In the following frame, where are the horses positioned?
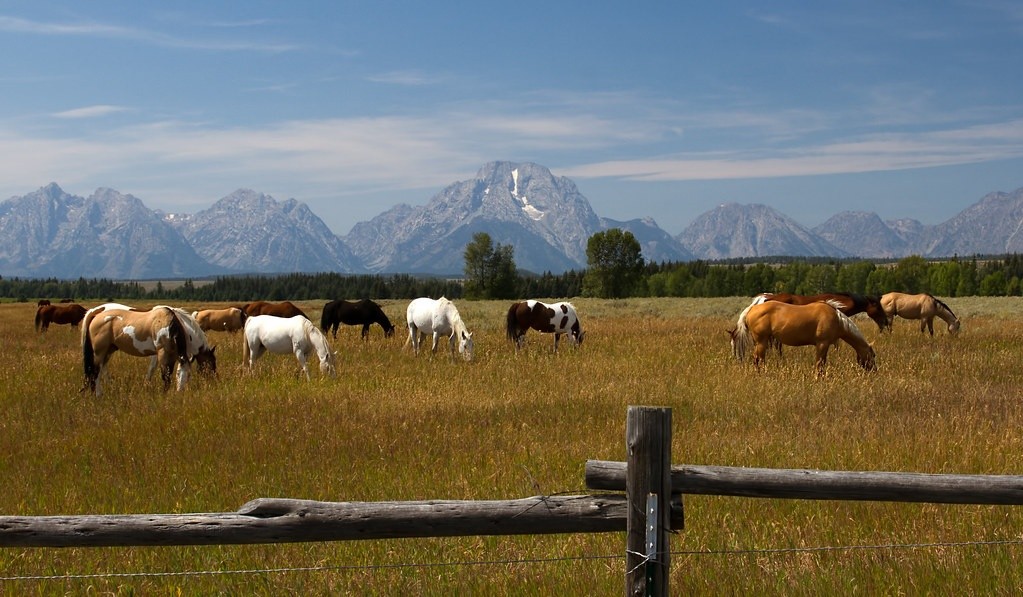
[34,299,395,396]
[507,299,586,352]
[403,297,473,361]
[728,291,961,374]
